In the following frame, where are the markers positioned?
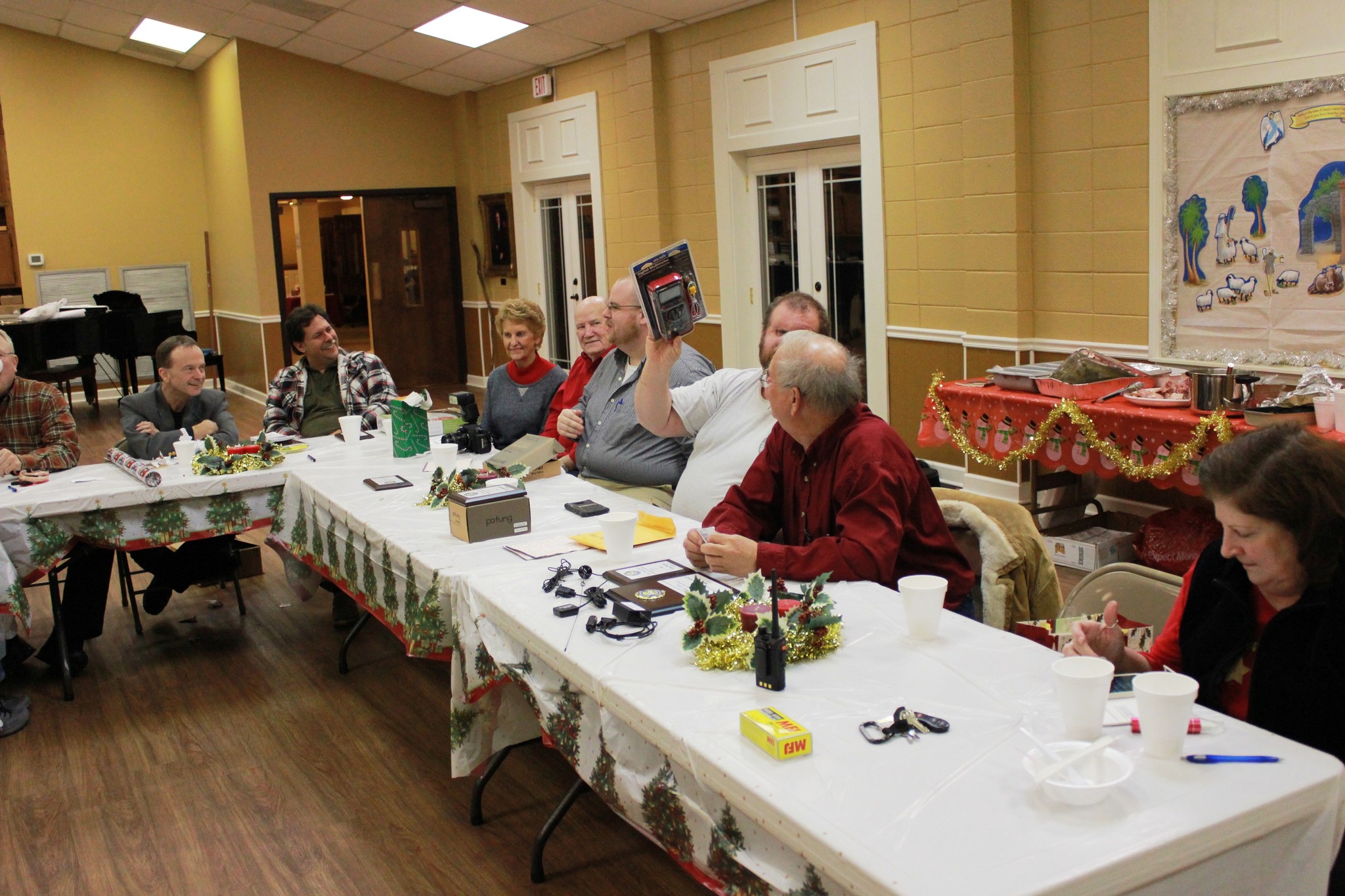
[1131,716,1215,734]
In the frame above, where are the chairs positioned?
[110,436,249,635]
[1057,560,1187,665]
[931,484,1063,645]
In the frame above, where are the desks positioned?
[918,372,1345,504]
[0,426,1345,894]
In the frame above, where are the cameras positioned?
[440,391,491,454]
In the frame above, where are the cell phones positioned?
[1107,671,1137,699]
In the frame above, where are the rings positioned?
[1107,620,1119,629]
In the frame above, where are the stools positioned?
[35,364,99,420]
[199,347,226,396]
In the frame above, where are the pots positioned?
[1185,369,1260,417]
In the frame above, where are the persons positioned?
[263,302,397,623]
[683,335,980,621]
[118,336,240,615]
[635,291,828,522]
[0,329,114,672]
[480,278,719,514]
[1062,420,1345,896]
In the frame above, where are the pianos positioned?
[0,290,197,413]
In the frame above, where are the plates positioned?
[1123,388,1191,407]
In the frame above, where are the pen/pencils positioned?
[1181,755,1284,764]
[307,455,316,462]
[8,486,18,492]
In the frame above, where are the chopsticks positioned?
[1033,737,1121,783]
[1015,724,1095,785]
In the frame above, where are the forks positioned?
[992,365,1004,371]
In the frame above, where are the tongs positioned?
[1091,382,1145,403]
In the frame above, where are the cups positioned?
[599,512,637,563]
[1132,671,1199,760]
[1313,389,1345,434]
[897,575,948,641]
[430,443,458,478]
[1051,656,1115,743]
[338,415,362,442]
[173,440,197,471]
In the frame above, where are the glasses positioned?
[607,303,641,313]
[759,370,807,398]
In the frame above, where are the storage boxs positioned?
[1040,524,1140,573]
[482,434,566,484]
[449,496,531,544]
[389,388,431,457]
[740,708,811,760]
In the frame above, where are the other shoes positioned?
[35,625,90,675]
[3,707,30,737]
[331,590,359,627]
[3,635,37,669]
[0,694,31,712]
[143,564,175,615]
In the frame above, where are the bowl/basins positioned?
[1024,741,1133,806]
